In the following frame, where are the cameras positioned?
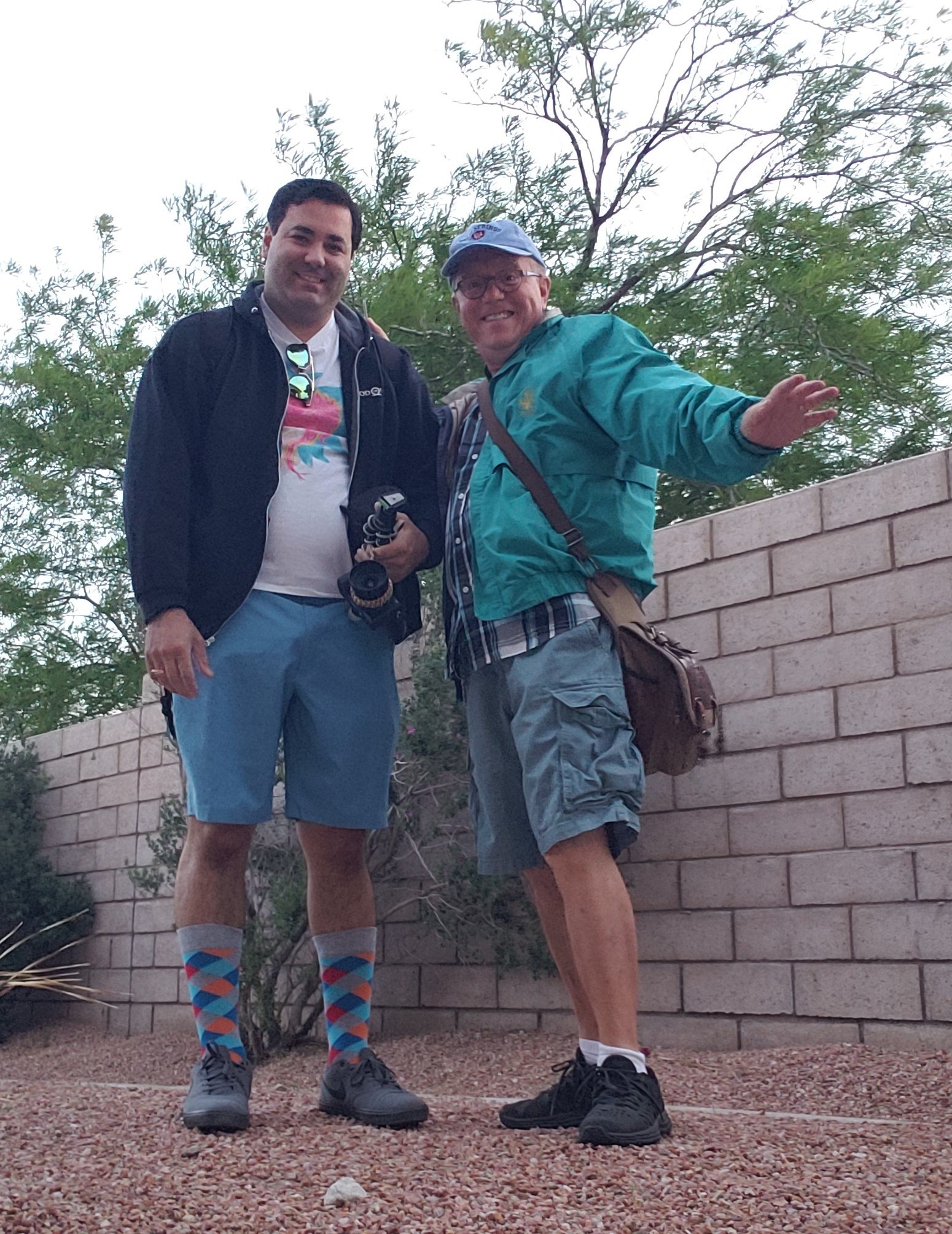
[337,560,410,628]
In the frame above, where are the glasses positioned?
[286,343,315,406]
[454,268,543,300]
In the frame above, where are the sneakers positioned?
[319,1048,429,1125]
[500,1047,599,1129]
[182,1040,253,1129]
[579,1055,672,1144]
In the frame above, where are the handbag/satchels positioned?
[614,622,724,775]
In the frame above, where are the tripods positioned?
[361,491,409,548]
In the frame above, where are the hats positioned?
[441,219,547,277]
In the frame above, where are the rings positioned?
[151,668,164,675]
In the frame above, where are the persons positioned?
[123,177,445,1132]
[367,220,841,1140]
